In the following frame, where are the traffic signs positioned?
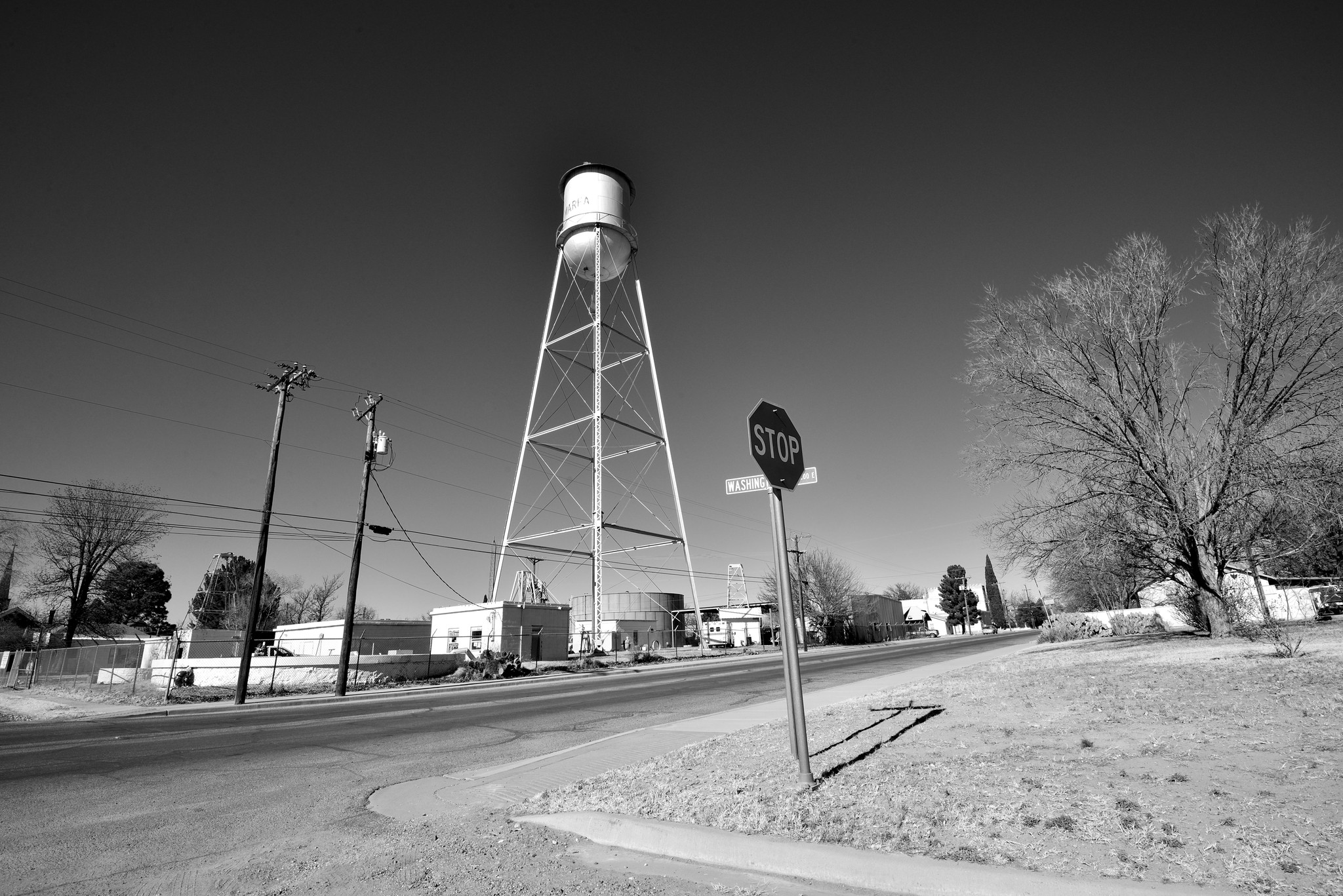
[726,466,819,494]
[1043,597,1055,605]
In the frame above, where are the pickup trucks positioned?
[906,626,939,639]
[252,645,314,657]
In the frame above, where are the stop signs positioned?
[746,397,805,492]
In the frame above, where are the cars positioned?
[982,625,998,635]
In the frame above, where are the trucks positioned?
[701,621,737,648]
